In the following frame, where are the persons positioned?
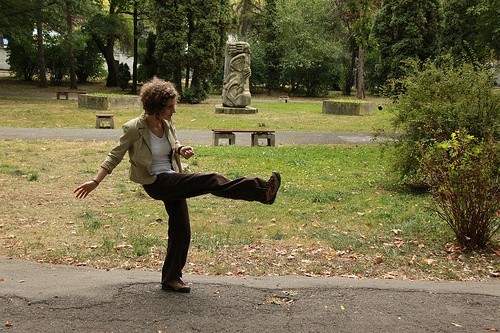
[68,75,282,293]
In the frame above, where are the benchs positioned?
[213,129,276,146]
[57,91,85,100]
[96,113,114,129]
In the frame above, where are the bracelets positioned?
[92,179,99,186]
[178,145,183,155]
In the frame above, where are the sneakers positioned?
[260,171,281,205]
[161,277,191,293]
